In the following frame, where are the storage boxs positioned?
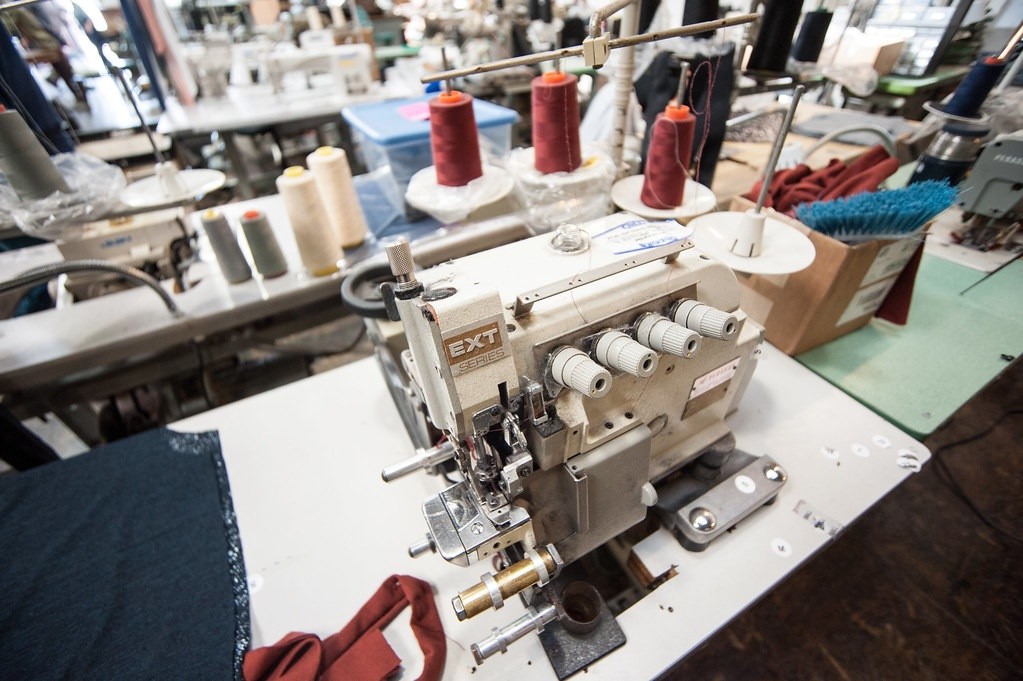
[341,92,519,224]
[56,203,192,263]
[838,29,908,77]
[731,191,932,359]
